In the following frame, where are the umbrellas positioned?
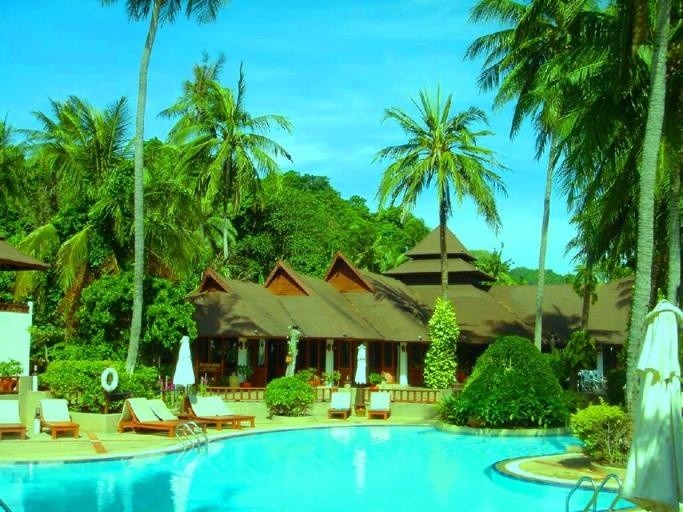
[620,300,682,511]
[354,344,367,385]
[172,335,195,395]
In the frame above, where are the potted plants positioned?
[0,359,24,394]
[235,364,255,391]
[367,372,387,390]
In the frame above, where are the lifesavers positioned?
[102,368,118,392]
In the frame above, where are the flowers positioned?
[286,325,309,356]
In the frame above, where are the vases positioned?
[284,356,292,363]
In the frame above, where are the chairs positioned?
[0,400,26,440]
[327,392,352,419]
[40,399,80,439]
[117,395,256,438]
[368,392,391,419]
